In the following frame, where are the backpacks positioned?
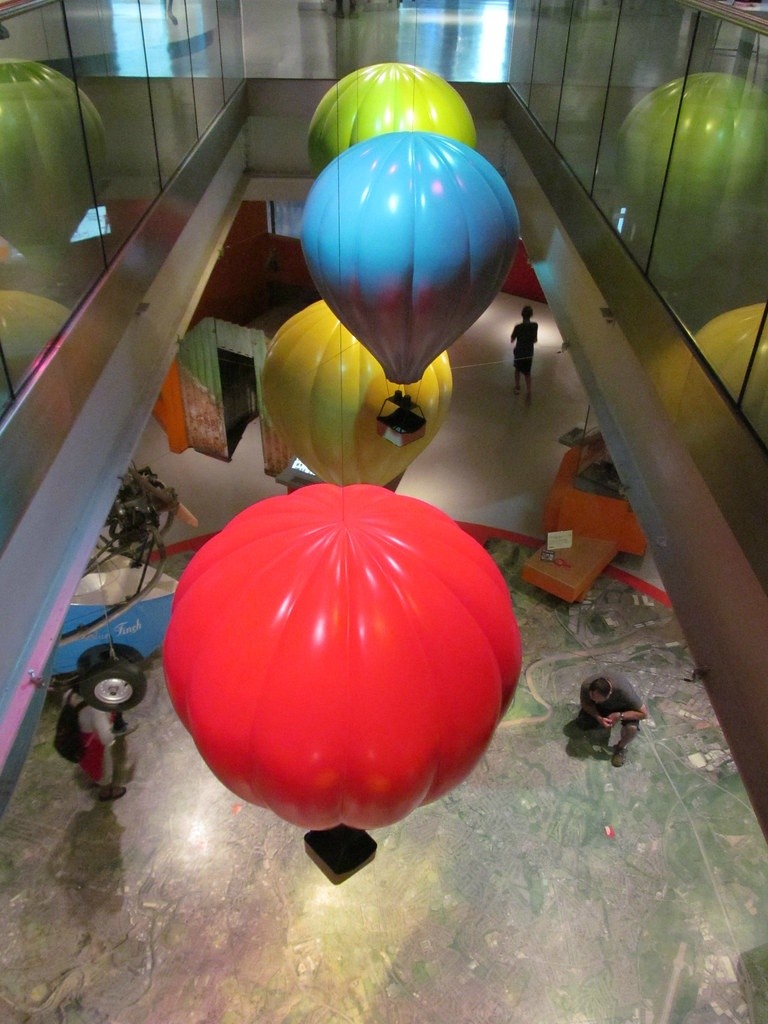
[53,689,88,763]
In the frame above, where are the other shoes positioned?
[611,741,627,767]
[513,386,520,394]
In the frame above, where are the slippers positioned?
[99,787,126,801]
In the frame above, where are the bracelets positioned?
[620,712,624,721]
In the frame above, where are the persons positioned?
[62,688,127,801]
[576,670,647,766]
[511,306,538,407]
[333,0,360,19]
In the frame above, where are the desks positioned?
[520,522,623,604]
